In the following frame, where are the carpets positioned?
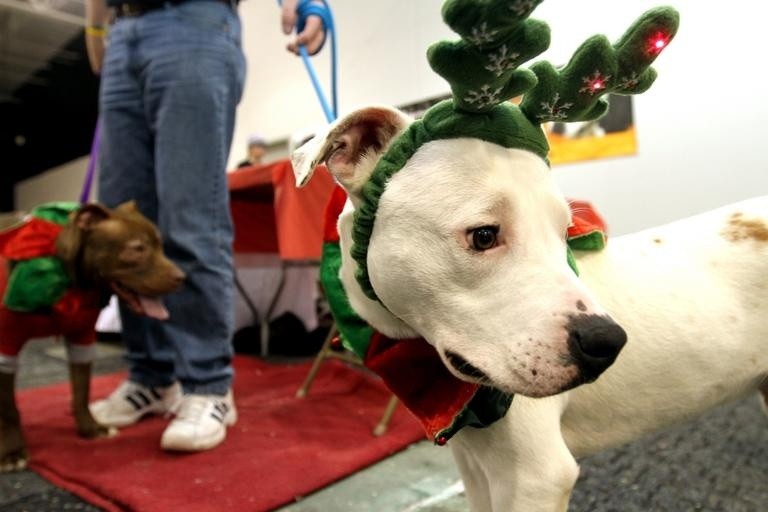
[13,352,427,512]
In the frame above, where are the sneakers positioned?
[161,387,239,452]
[88,379,182,427]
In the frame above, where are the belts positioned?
[115,0,188,16]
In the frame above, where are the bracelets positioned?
[84,20,107,36]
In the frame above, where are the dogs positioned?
[289,104,768,512]
[1,196,191,475]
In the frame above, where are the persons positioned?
[84,0,325,456]
